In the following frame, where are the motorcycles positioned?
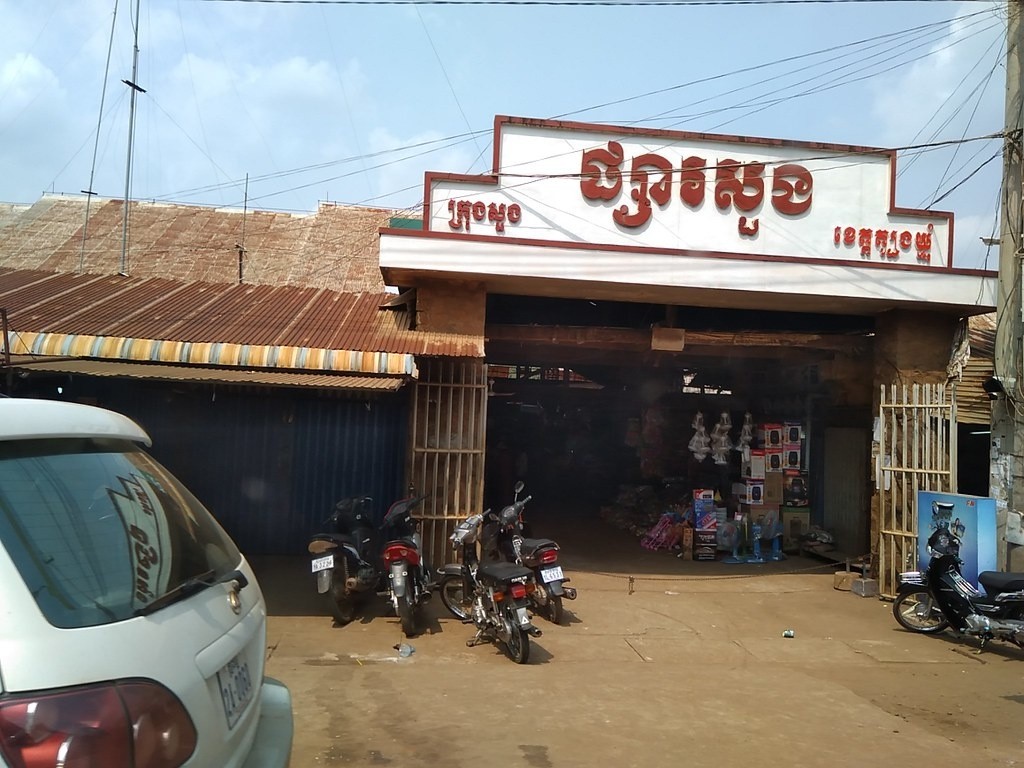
[307,492,384,624]
[488,481,578,623]
[376,492,437,636]
[437,507,542,664]
[893,501,1024,655]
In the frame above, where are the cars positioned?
[1,398,294,768]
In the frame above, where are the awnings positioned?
[0,330,419,393]
